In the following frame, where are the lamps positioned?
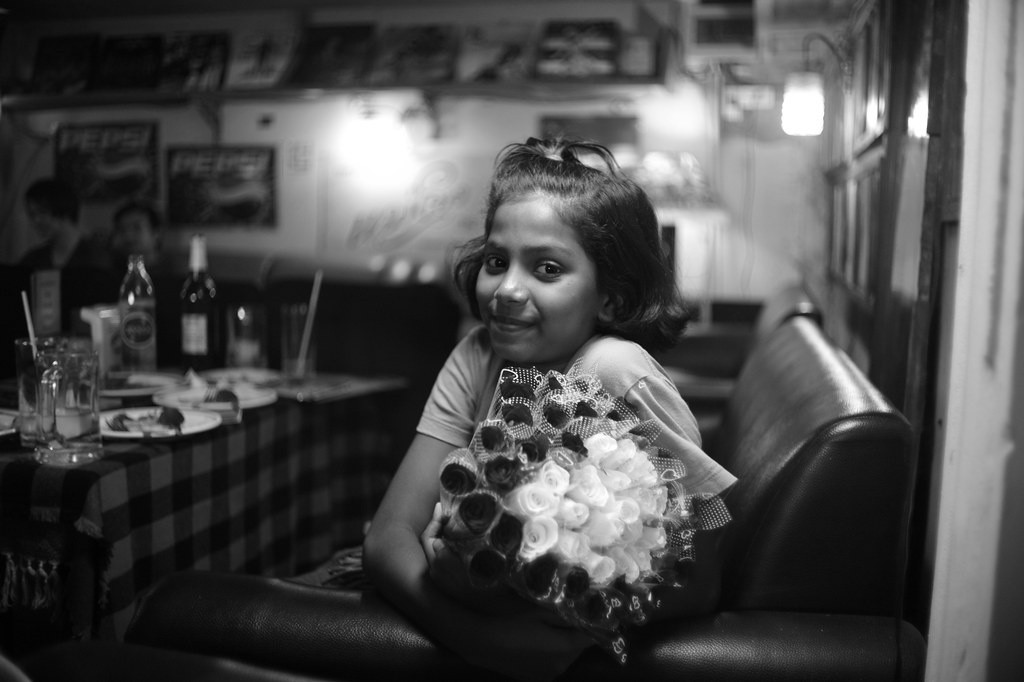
[780,32,852,138]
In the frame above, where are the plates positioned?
[152,385,278,411]
[200,366,282,385]
[98,369,183,396]
[101,406,222,439]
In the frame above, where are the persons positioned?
[360,137,740,682]
[113,203,187,366]
[16,174,113,330]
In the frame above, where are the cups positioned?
[34,348,104,467]
[283,306,315,381]
[14,337,70,446]
[228,308,268,368]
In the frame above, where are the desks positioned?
[1,375,394,667]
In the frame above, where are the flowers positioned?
[320,363,732,668]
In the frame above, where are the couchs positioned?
[103,288,916,681]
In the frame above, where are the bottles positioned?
[119,254,157,374]
[179,232,220,379]
